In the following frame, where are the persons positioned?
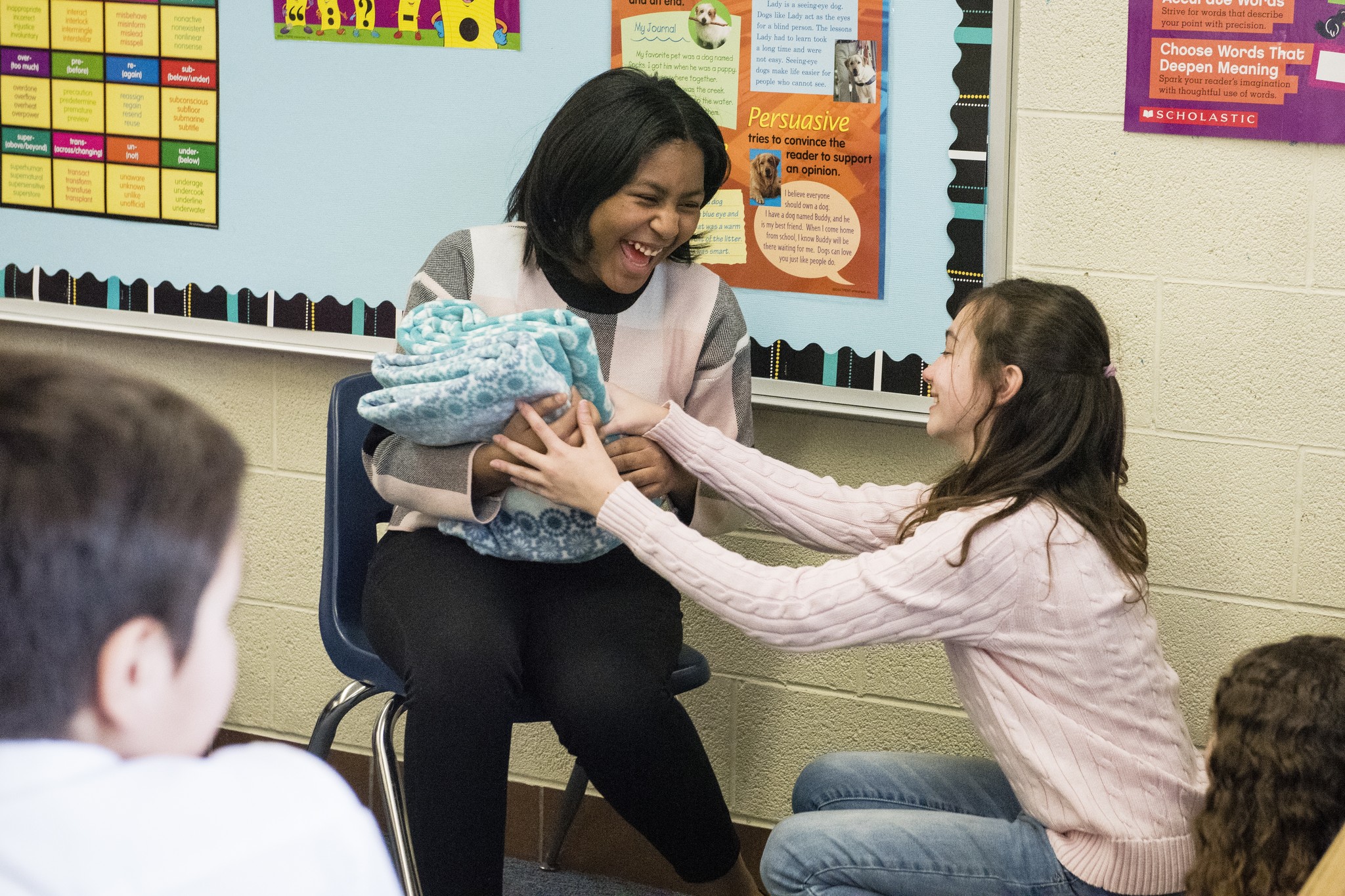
[487,279,1205,896]
[1185,634,1345,896]
[359,65,760,896]
[1,350,407,895]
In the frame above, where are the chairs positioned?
[308,372,712,896]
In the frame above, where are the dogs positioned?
[844,54,876,104]
[749,153,782,204]
[694,3,728,50]
